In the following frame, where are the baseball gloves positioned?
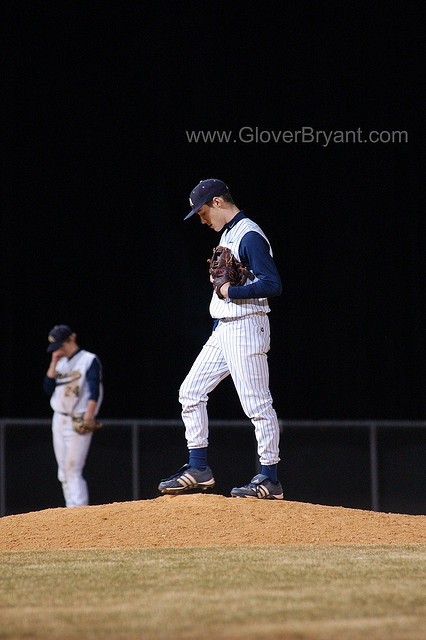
[208,246,255,300]
[74,413,102,434]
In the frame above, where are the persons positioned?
[158,180,284,500]
[47,325,104,507]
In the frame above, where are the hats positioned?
[183,179,225,220]
[46,325,70,351]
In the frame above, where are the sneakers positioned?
[157,464,214,493]
[230,474,283,500]
